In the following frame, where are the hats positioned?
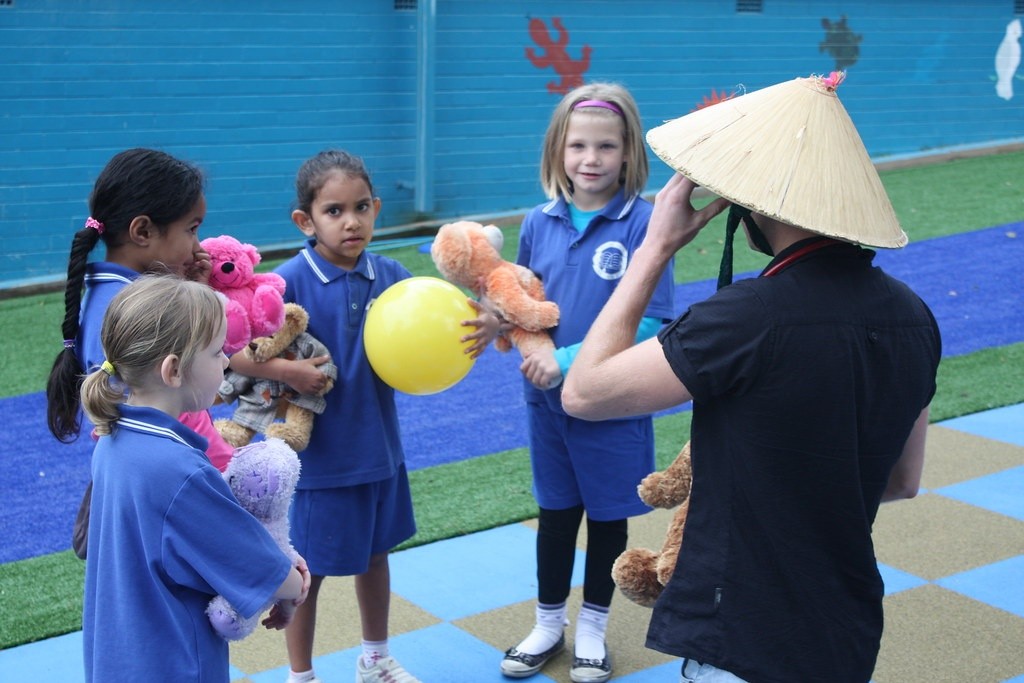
[643,70,913,250]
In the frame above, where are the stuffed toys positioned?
[611,438,693,610]
[205,439,300,641]
[199,235,290,356]
[431,220,560,363]
[211,302,337,452]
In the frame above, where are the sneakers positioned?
[356,656,423,683]
[287,676,322,683]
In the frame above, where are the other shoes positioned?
[566,626,612,681]
[494,625,567,681]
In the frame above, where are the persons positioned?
[46,151,237,560]
[501,82,676,683]
[561,72,942,683]
[79,275,311,683]
[268,153,421,683]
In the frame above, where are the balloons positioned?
[363,276,477,396]
